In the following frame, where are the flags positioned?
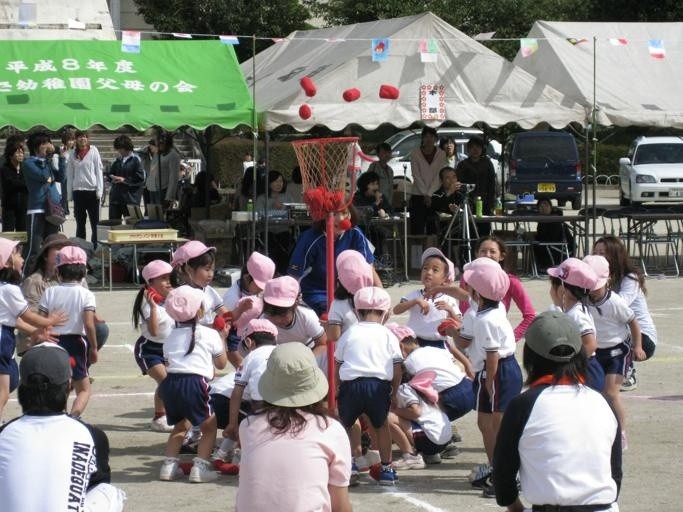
[473,31,496,39]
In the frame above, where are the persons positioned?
[34,247,99,419]
[367,143,394,204]
[353,172,394,258]
[1,142,28,258]
[0,236,68,421]
[484,133,502,174]
[0,343,126,512]
[535,197,577,276]
[104,136,145,224]
[258,276,328,349]
[335,286,406,485]
[456,136,496,237]
[427,168,474,234]
[235,341,353,512]
[214,252,276,338]
[388,365,452,469]
[26,133,62,255]
[45,132,70,220]
[460,238,536,343]
[387,323,474,458]
[139,124,162,215]
[490,312,620,512]
[412,127,446,235]
[286,166,304,198]
[223,318,280,465]
[327,249,382,470]
[17,236,109,368]
[180,172,209,217]
[157,285,228,483]
[1,135,25,168]
[179,366,253,476]
[393,248,464,442]
[549,258,604,394]
[256,172,297,273]
[67,130,104,248]
[582,255,646,453]
[463,264,523,497]
[258,158,266,170]
[143,129,180,218]
[439,136,469,168]
[478,133,502,163]
[201,124,228,204]
[311,312,331,379]
[239,166,264,210]
[243,152,255,171]
[135,259,175,432]
[286,196,384,322]
[346,132,379,177]
[170,240,253,454]
[593,236,657,391]
[426,258,507,482]
[60,133,75,219]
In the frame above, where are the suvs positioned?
[618,136,682,211]
[359,129,504,193]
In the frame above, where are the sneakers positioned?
[151,416,176,431]
[392,454,425,470]
[442,446,459,459]
[380,468,400,486]
[189,464,220,482]
[354,456,369,471]
[349,463,360,487]
[181,431,235,464]
[468,463,523,497]
[620,367,637,390]
[621,431,627,452]
[160,461,186,481]
[424,453,441,464]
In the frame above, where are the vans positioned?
[505,131,582,210]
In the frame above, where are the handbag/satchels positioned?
[45,162,66,227]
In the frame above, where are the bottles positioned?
[496,198,502,216]
[476,197,482,218]
[247,199,253,211]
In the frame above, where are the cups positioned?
[566,201,572,209]
[525,194,535,202]
[551,199,558,206]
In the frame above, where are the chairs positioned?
[602,207,645,267]
[135,219,178,285]
[579,208,610,256]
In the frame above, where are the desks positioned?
[623,211,682,269]
[95,234,187,293]
[439,210,585,266]
[269,210,406,286]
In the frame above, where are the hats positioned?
[421,247,454,282]
[264,276,301,307]
[258,342,328,407]
[409,369,440,403]
[463,266,510,301]
[336,250,374,294]
[583,255,610,290]
[391,325,417,342]
[36,233,79,259]
[19,341,73,389]
[547,257,597,289]
[525,310,583,361]
[55,246,87,266]
[142,260,174,281]
[231,296,264,336]
[353,287,391,311]
[247,252,275,289]
[175,240,217,264]
[165,285,203,322]
[0,237,20,270]
[463,257,502,271]
[237,319,278,356]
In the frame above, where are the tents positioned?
[241,12,590,259]
[0,28,259,218]
[511,21,683,255]
